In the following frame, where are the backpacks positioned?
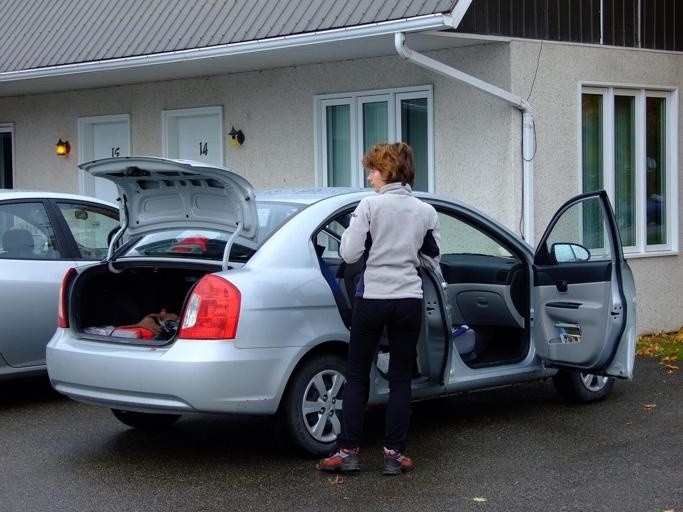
[109,308,178,339]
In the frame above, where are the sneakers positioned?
[383,446,415,474]
[319,446,362,471]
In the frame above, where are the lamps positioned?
[227,125,245,150]
[54,137,70,156]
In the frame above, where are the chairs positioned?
[0,229,38,259]
[343,249,476,378]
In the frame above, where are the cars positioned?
[0,189,119,384]
[45,155,638,458]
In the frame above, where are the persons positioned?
[315,143,441,475]
